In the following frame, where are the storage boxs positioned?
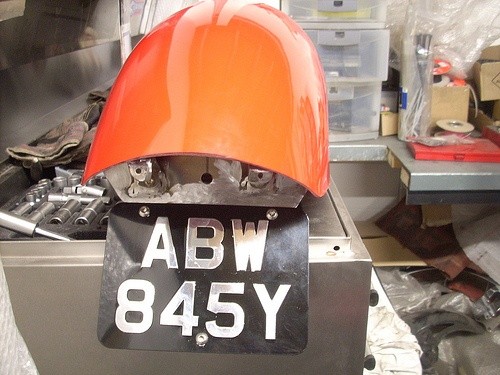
[280,0,500,163]
